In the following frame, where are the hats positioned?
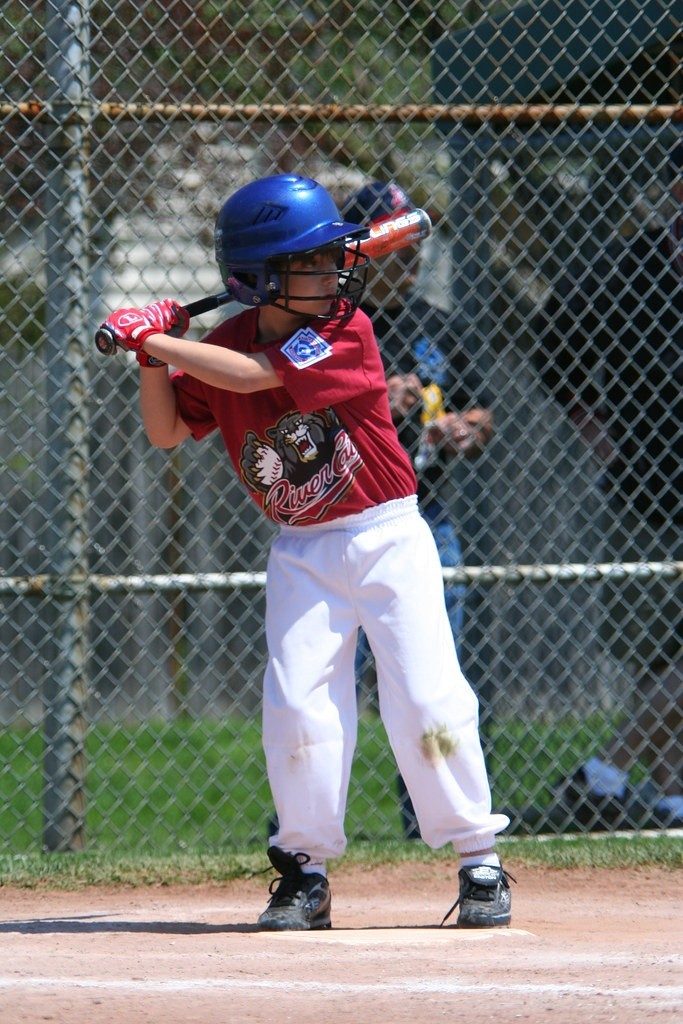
[340,181,439,238]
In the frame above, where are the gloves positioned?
[96,308,164,356]
[135,299,189,368]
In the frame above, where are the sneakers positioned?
[243,846,332,931]
[439,861,517,930]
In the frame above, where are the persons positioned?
[101,177,511,928]
[269,143,682,841]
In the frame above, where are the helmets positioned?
[213,174,370,307]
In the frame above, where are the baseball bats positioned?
[94,205,436,356]
